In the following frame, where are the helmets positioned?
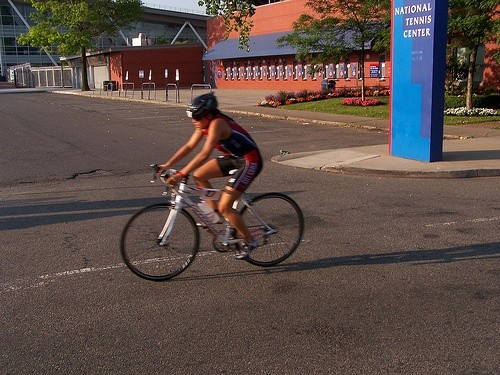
[185,92,219,119]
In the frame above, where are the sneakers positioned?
[195,210,225,226]
[233,236,260,259]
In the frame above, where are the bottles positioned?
[199,200,220,223]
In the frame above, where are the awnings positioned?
[202,19,390,63]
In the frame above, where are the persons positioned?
[156,93,262,259]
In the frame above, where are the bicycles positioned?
[120,163,304,281]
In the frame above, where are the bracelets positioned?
[177,172,184,178]
